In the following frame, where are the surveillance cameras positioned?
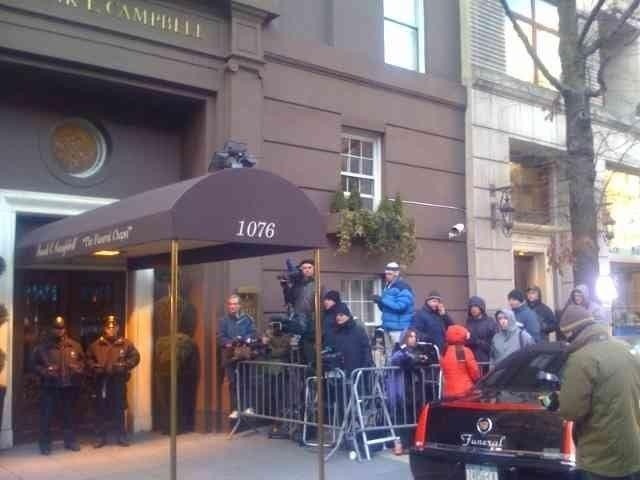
[448,223,466,239]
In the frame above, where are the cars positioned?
[411,334,640,480]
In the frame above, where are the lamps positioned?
[489,182,515,239]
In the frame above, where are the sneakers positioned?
[63,442,81,452]
[116,439,131,448]
[227,410,240,420]
[243,407,256,417]
[92,440,107,449]
[39,446,52,456]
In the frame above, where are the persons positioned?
[86,311,140,448]
[29,314,85,454]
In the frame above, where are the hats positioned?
[558,304,595,333]
[423,289,441,299]
[323,290,340,305]
[101,314,122,328]
[384,262,401,277]
[297,258,315,267]
